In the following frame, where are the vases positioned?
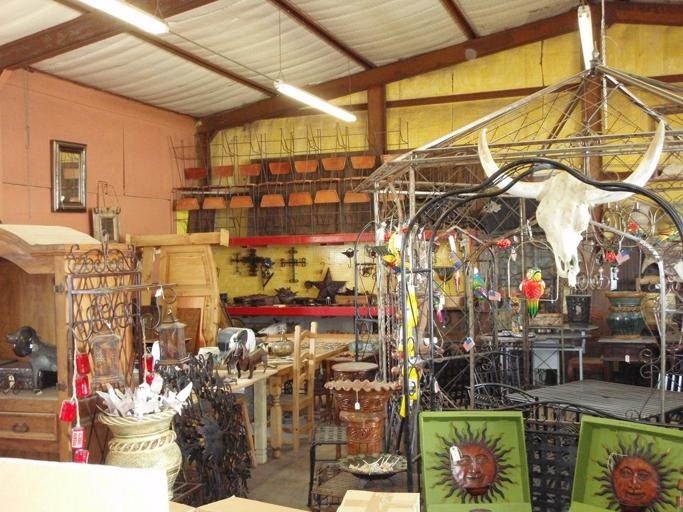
[605,290,646,339]
[641,293,675,335]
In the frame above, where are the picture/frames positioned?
[50,138,87,212]
[92,207,120,242]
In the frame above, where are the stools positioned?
[569,357,606,382]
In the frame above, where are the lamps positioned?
[94,322,124,383]
[158,308,190,365]
[78,1,168,36]
[578,5,594,68]
[273,79,355,123]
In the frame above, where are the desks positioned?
[213,369,280,463]
[310,470,423,507]
[600,336,656,382]
[256,341,349,459]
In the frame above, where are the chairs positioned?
[638,348,683,392]
[466,381,538,420]
[168,120,406,210]
[307,389,399,507]
[474,350,522,408]
[267,322,318,450]
[313,393,406,512]
